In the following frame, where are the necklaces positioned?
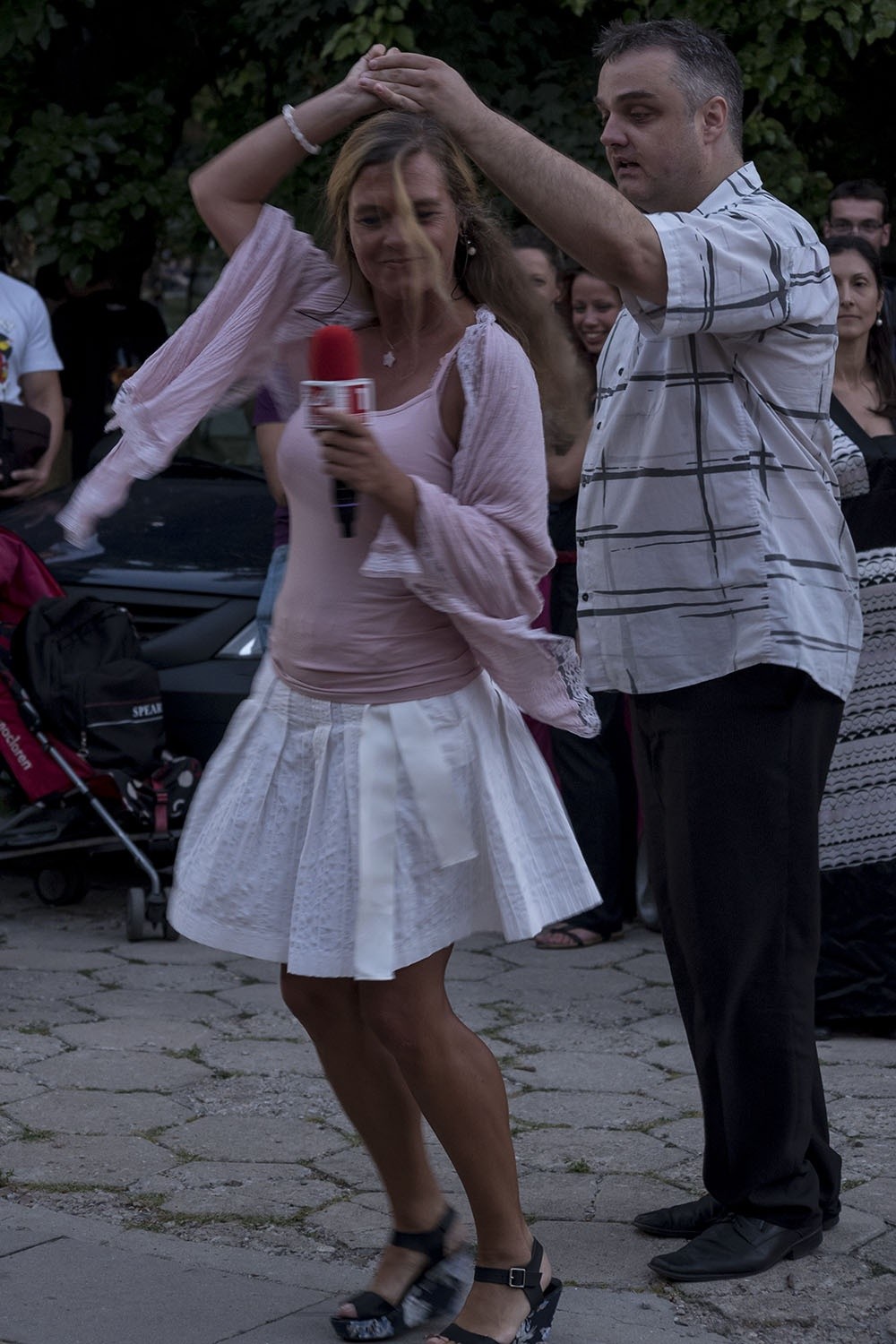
[381,322,429,367]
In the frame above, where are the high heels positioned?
[425,1236,562,1344]
[328,1199,477,1344]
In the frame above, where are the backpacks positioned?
[18,592,166,774]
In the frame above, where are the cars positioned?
[63,432,278,769]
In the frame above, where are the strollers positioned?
[0,526,224,941]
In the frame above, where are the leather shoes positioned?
[648,1212,825,1282]
[632,1192,840,1238]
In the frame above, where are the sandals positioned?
[534,924,624,949]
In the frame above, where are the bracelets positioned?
[282,104,321,155]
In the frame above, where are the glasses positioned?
[827,220,884,236]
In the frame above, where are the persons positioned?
[498,174,896,1044]
[357,20,864,1278]
[50,44,606,1344]
[0,270,63,499]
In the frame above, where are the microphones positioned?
[304,323,378,537]
